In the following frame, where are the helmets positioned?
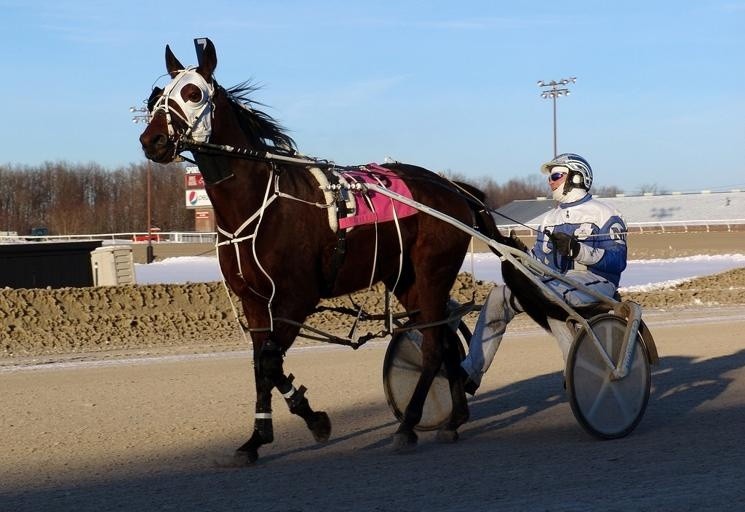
[540,152,593,191]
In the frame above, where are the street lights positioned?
[537,76,576,157]
[130,100,153,263]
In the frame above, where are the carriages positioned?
[139,38,659,464]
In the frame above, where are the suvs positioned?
[28,227,47,241]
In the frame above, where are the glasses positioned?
[548,172,567,182]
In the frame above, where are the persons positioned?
[454,153,627,397]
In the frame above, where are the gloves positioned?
[551,231,581,260]
[510,229,527,253]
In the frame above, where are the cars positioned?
[134,233,158,241]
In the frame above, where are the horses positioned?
[139,37,553,467]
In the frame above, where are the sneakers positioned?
[458,369,479,396]
